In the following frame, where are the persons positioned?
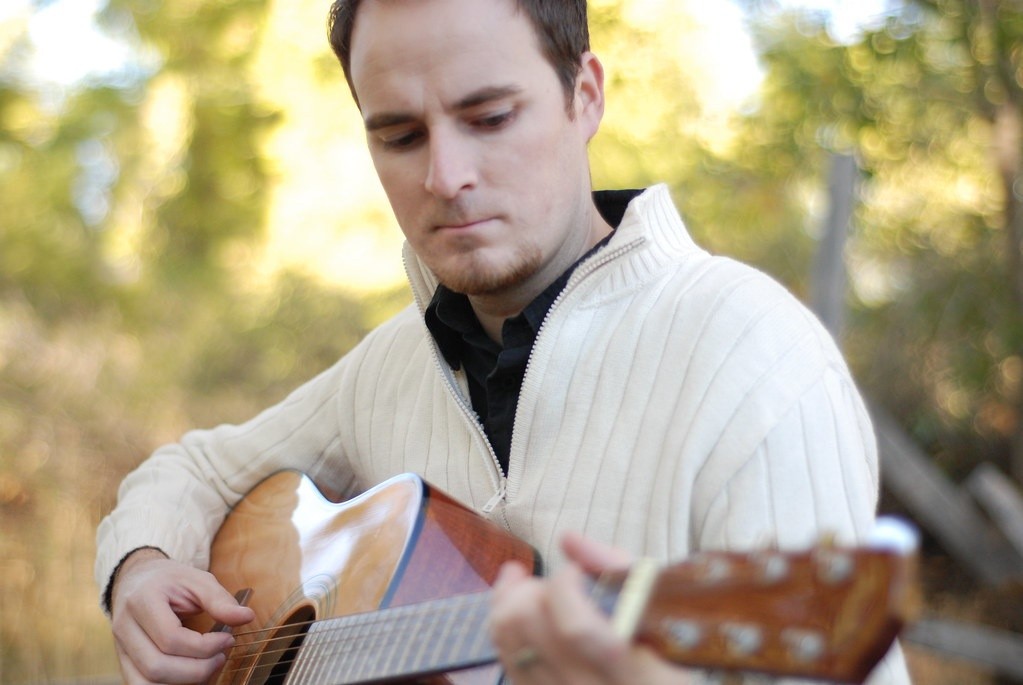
[95,1,915,685]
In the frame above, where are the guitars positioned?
[179,468,922,685]
